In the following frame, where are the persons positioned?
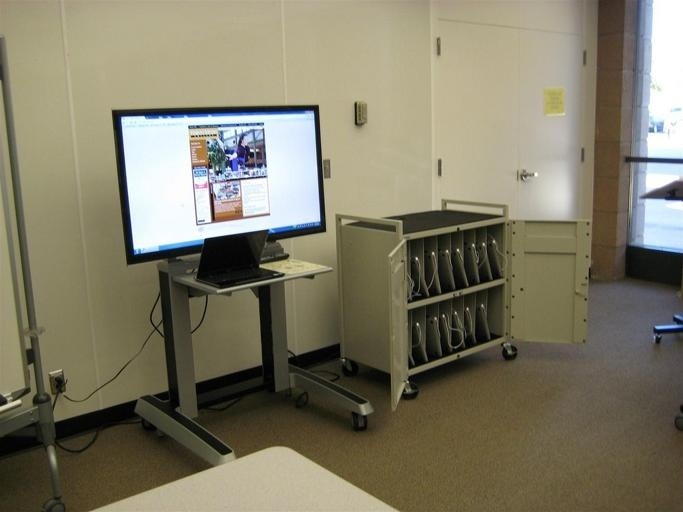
[228,140,239,171]
[237,133,250,167]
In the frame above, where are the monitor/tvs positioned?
[111,104,326,289]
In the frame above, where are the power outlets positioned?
[48,368,66,395]
